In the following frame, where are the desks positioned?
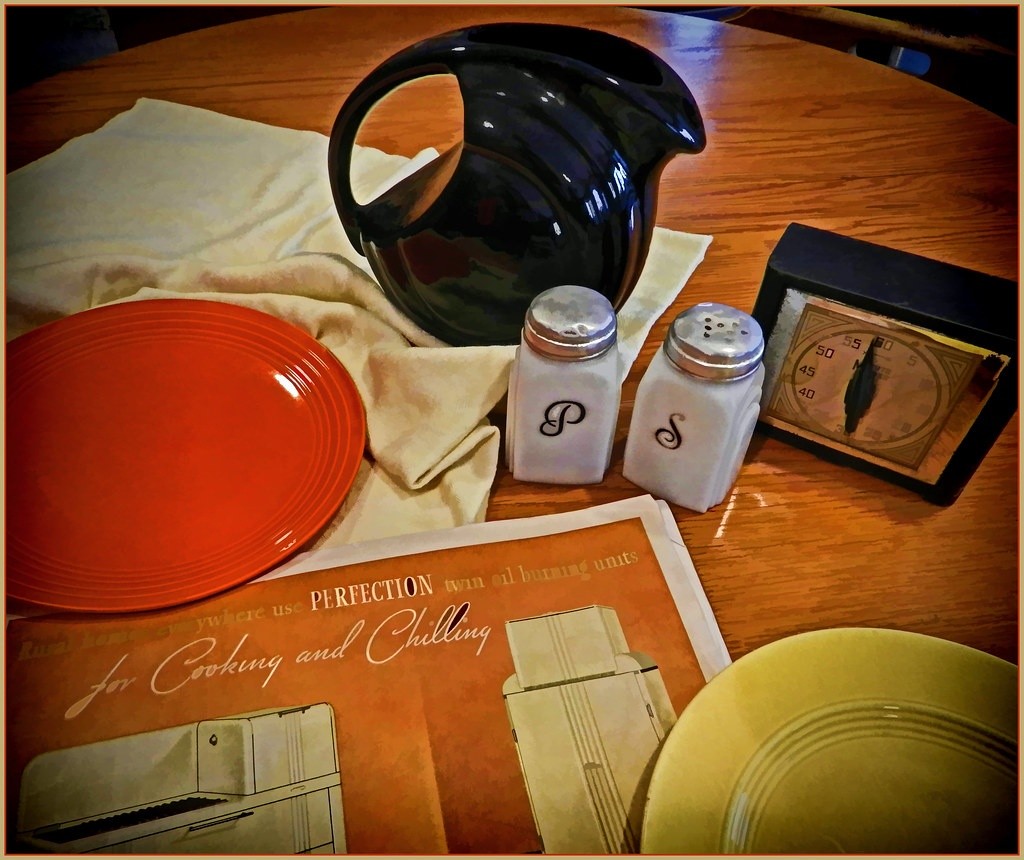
[6,7,1021,855]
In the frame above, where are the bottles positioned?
[623,297,766,512]
[505,286,624,486]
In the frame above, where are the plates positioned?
[5,300,365,614]
[635,629,1022,858]
